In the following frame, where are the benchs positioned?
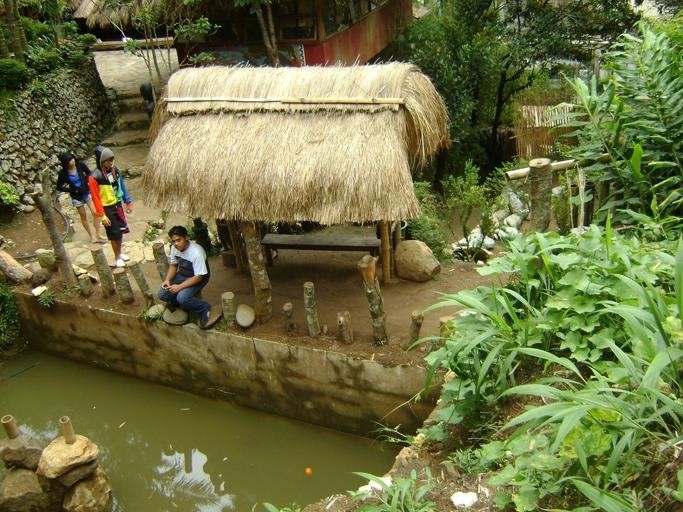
[261,232,382,269]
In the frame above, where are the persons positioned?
[158,226,210,326]
[57,152,107,243]
[89,146,132,267]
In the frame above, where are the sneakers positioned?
[114,251,130,268]
[199,304,212,327]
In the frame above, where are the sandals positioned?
[90,236,107,244]
